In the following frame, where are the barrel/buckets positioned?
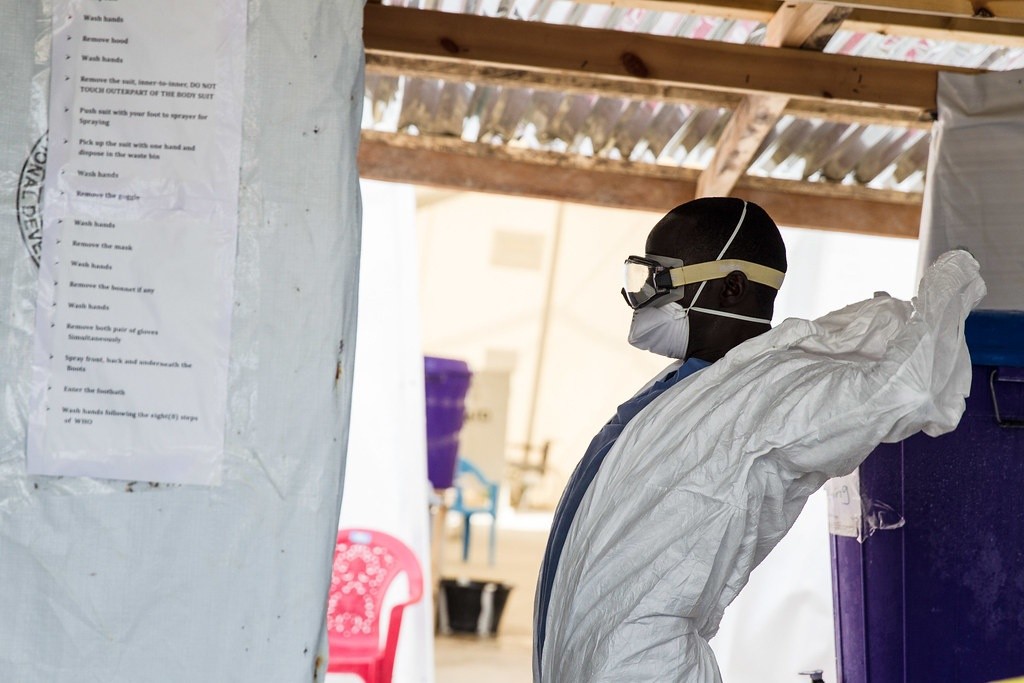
[436,578,513,637]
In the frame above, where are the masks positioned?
[627,281,726,360]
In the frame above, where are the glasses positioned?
[621,255,673,309]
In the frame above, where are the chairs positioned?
[433,459,498,565]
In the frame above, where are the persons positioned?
[533,196,986,683]
